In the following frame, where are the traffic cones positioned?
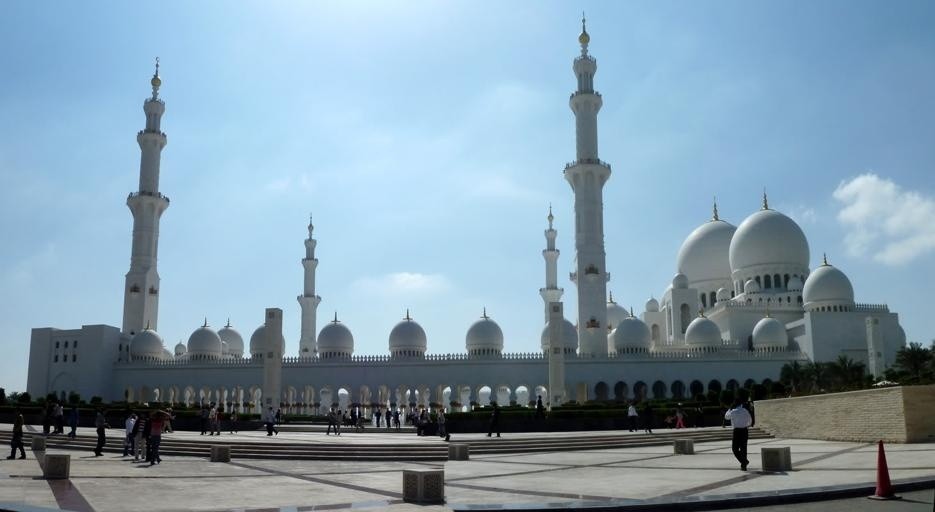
[867,438,903,501]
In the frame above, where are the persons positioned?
[93,409,111,456]
[6,410,25,459]
[694,403,707,427]
[487,399,502,436]
[198,404,239,438]
[627,403,639,431]
[267,407,278,435]
[676,403,687,428]
[276,408,281,426]
[725,399,753,470]
[326,404,448,438]
[122,406,177,465]
[43,397,79,438]
